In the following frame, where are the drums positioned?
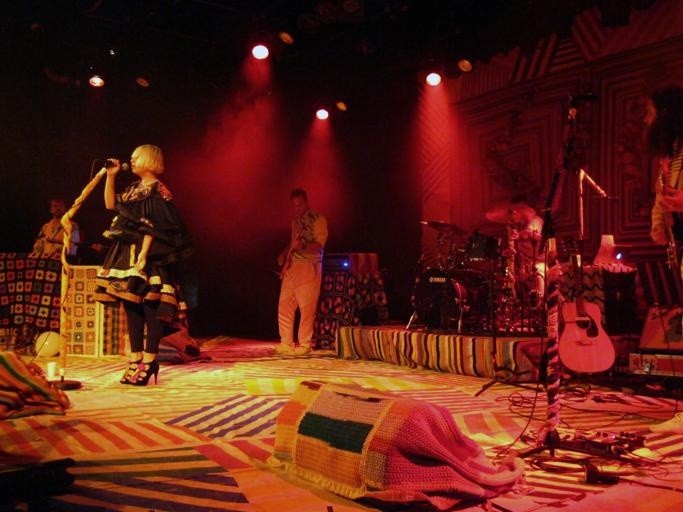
[453,234,498,272]
[490,270,544,312]
[414,268,488,328]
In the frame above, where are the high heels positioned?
[119,354,159,386]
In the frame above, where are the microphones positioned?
[582,169,607,198]
[106,160,128,171]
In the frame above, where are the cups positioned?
[47,362,57,379]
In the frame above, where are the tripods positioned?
[509,239,616,458]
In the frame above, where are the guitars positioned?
[276,227,304,280]
[559,238,616,375]
[652,173,682,276]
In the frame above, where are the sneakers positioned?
[265,344,313,358]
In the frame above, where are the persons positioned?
[507,194,561,310]
[276,188,329,357]
[92,144,180,388]
[27,197,79,260]
[642,85,683,258]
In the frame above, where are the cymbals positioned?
[486,211,513,223]
[428,220,466,235]
